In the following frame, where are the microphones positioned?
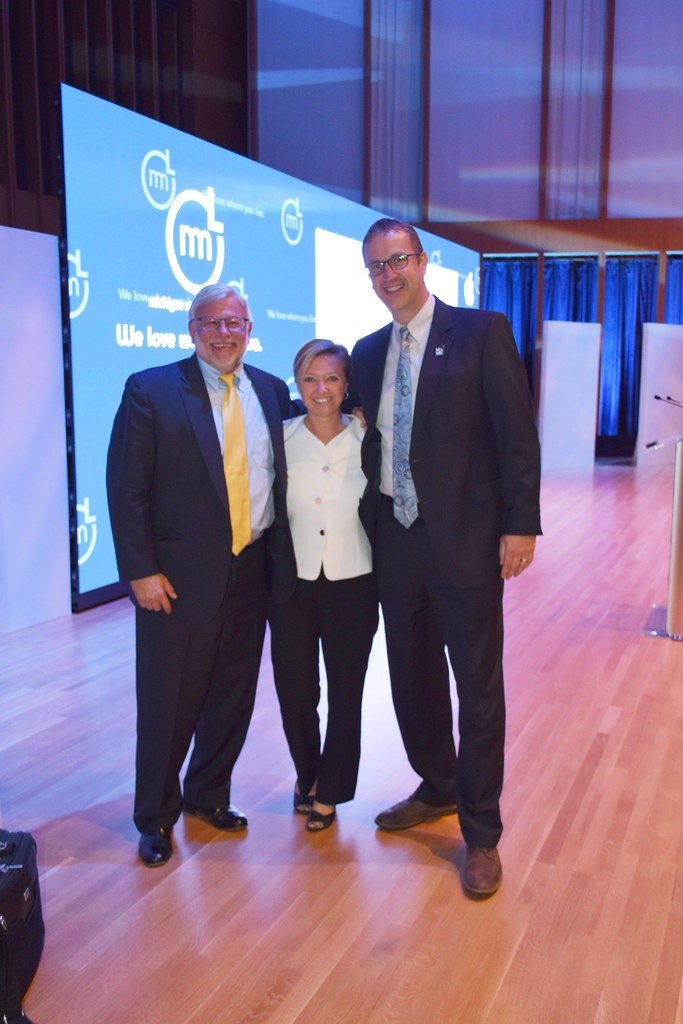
[654,395,683,410]
[666,396,683,405]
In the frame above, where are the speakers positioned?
[0,827,45,1024]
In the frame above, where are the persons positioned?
[266,338,383,830]
[104,283,367,869]
[343,218,544,899]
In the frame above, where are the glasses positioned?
[193,315,251,331]
[365,253,422,277]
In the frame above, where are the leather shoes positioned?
[463,843,503,895]
[374,793,459,830]
[138,823,172,866]
[183,797,249,831]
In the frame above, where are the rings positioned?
[521,558,527,562]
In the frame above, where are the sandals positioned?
[293,779,318,812]
[306,799,336,831]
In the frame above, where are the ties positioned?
[218,374,252,556]
[393,327,419,529]
